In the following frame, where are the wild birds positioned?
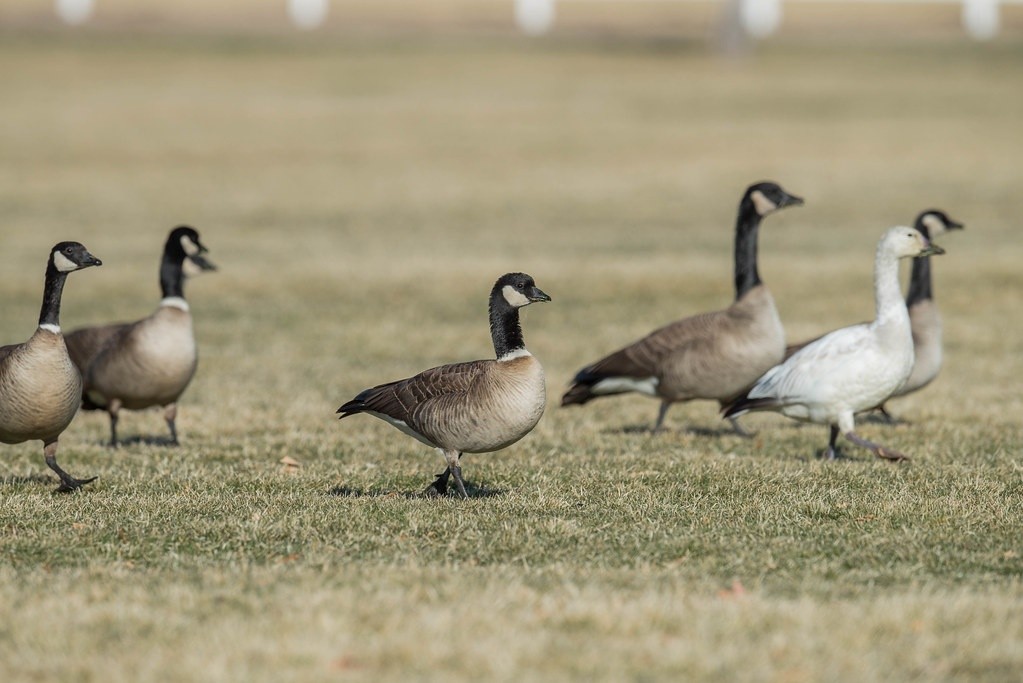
[717,226,946,463]
[0,241,103,494]
[781,207,964,423]
[558,180,804,438]
[335,271,551,503]
[63,226,218,452]
